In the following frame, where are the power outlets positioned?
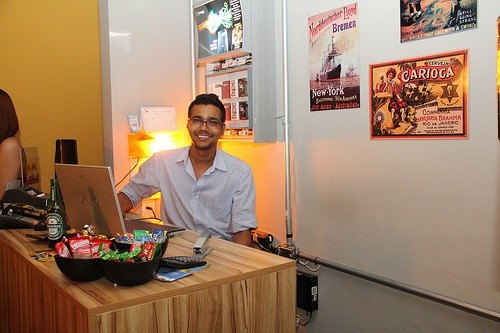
[142,200,155,217]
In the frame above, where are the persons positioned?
[117,94,258,247]
[0,89,26,199]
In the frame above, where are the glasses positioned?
[189,118,224,127]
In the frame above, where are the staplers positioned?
[193,230,214,260]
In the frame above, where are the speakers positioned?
[54,139,78,179]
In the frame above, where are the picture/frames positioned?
[203,67,253,130]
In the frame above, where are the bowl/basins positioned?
[54,233,169,287]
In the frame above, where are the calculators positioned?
[162,255,206,269]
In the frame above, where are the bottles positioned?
[46,178,67,248]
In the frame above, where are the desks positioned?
[0,195,297,333]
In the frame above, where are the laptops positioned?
[53,163,186,239]
